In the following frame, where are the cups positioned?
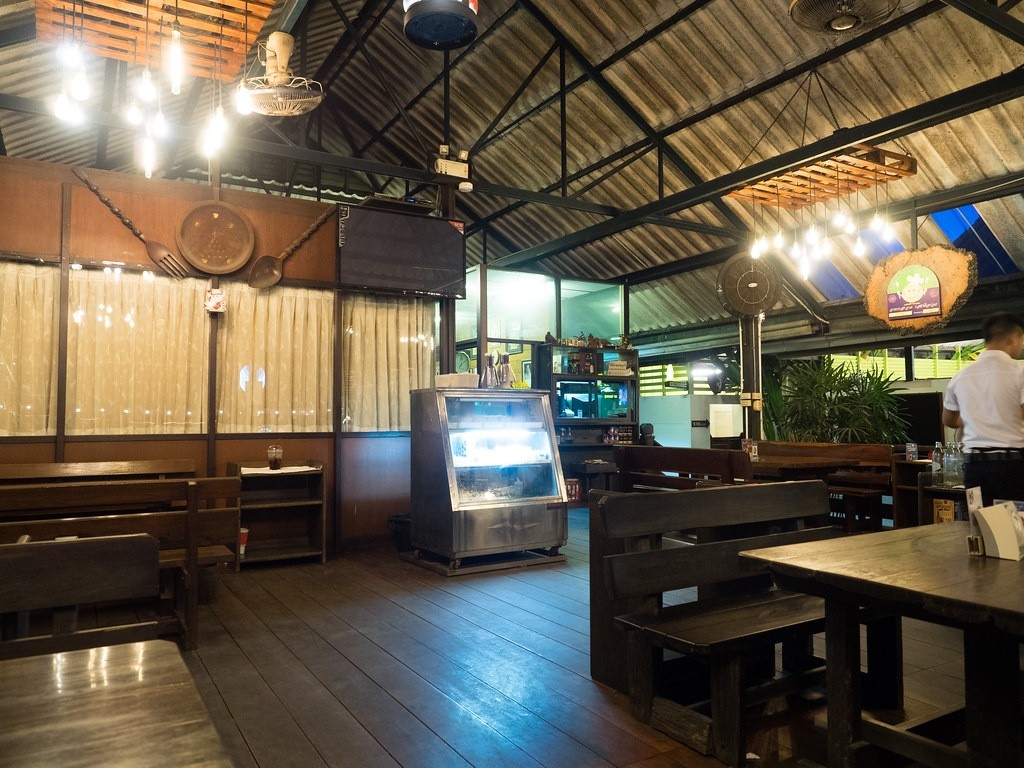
[267,444,283,470]
[906,443,918,461]
[239,528,249,555]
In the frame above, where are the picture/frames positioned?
[505,319,522,354]
[521,360,531,389]
[470,325,477,359]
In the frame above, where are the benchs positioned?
[0,459,241,661]
[590,441,895,768]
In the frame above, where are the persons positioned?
[942,314,1024,508]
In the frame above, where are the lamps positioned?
[728,71,917,283]
[435,145,468,178]
[35,0,276,179]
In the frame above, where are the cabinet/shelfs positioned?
[542,346,639,509]
[891,453,965,529]
[227,460,327,572]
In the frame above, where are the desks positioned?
[751,455,859,481]
[739,521,1024,768]
[0,639,234,768]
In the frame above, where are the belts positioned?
[963,451,1024,463]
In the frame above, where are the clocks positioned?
[456,351,470,373]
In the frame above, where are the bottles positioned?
[931,441,964,488]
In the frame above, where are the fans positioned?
[716,251,781,319]
[240,31,324,117]
[788,0,900,34]
